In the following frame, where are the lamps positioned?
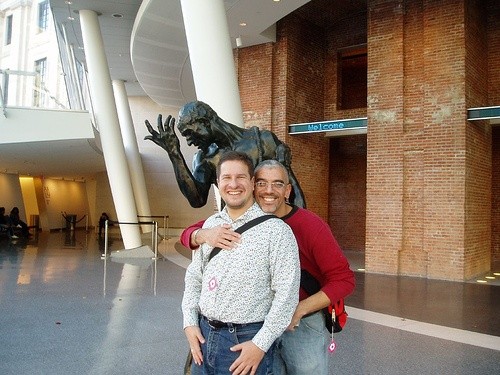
[235,35,242,48]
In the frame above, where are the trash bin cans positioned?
[30,215,39,226]
[66,215,77,230]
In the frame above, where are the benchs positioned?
[95,225,121,240]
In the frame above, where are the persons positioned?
[98,212,109,236]
[143,101,307,209]
[180,159,356,375]
[0,206,32,242]
[180,148,300,375]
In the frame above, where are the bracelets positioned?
[194,229,201,244]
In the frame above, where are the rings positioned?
[190,348,194,352]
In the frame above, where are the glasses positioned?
[255,181,288,189]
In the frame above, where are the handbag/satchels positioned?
[324,298,348,333]
[183,351,193,375]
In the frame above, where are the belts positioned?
[204,317,236,330]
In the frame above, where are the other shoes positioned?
[8,240,17,244]
[11,235,18,239]
